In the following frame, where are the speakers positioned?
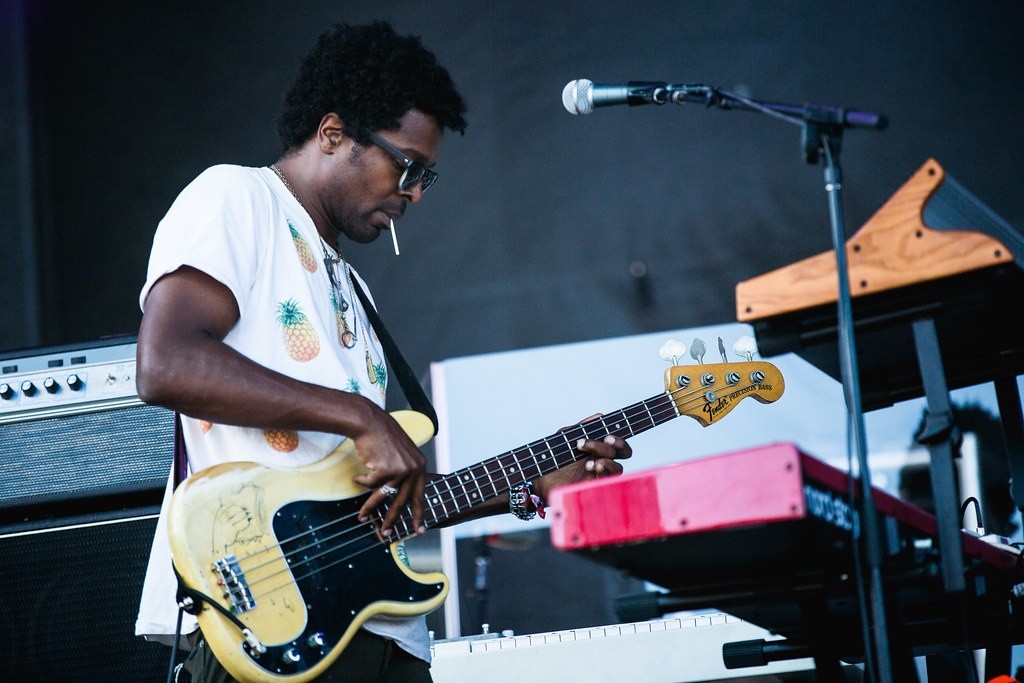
[0,500,187,683]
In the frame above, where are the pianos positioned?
[551,442,1023,683]
[424,603,817,683]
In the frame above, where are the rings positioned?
[380,484,398,497]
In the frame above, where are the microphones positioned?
[562,79,717,116]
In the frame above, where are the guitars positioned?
[163,334,786,683]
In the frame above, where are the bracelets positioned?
[509,481,545,521]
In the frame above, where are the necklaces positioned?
[270,165,367,349]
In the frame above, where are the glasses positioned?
[363,128,440,196]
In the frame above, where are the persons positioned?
[134,18,631,683]
